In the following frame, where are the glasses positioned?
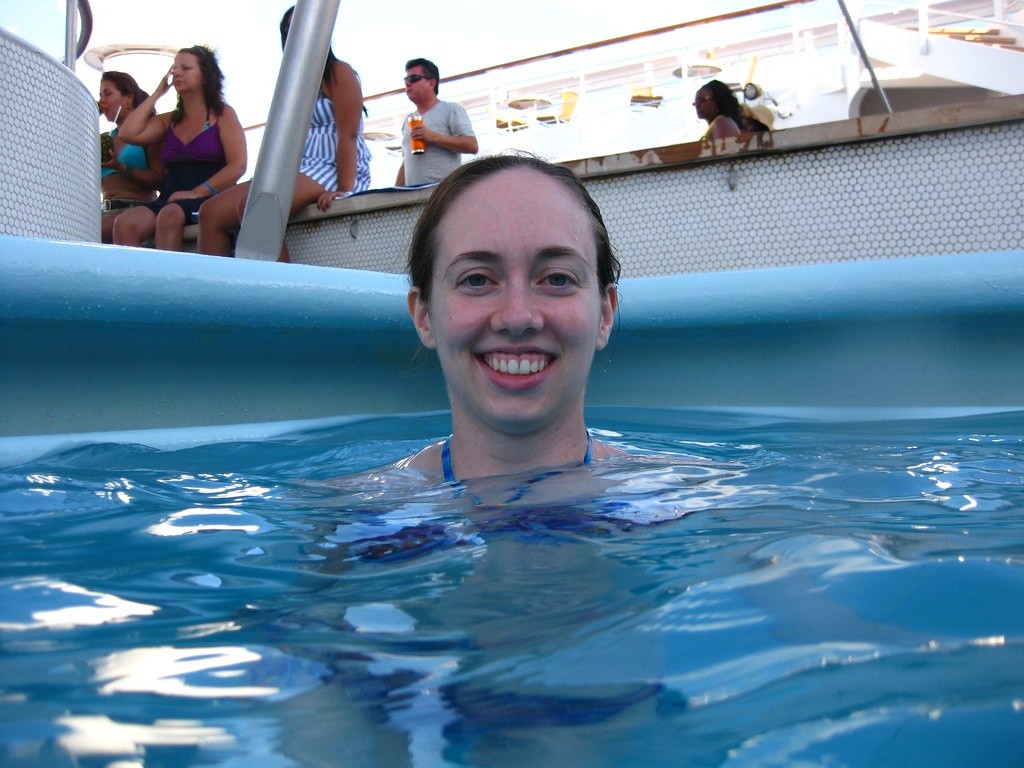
[404,73,433,84]
[694,96,715,104]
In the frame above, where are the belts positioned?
[101,199,147,212]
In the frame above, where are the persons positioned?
[198,5,372,263]
[395,58,478,186]
[400,149,622,484]
[97,70,158,245]
[692,79,774,140]
[112,45,247,253]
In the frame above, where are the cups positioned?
[408,116,425,155]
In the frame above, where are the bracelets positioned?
[120,163,139,182]
[203,182,217,195]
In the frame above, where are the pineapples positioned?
[101,134,114,162]
